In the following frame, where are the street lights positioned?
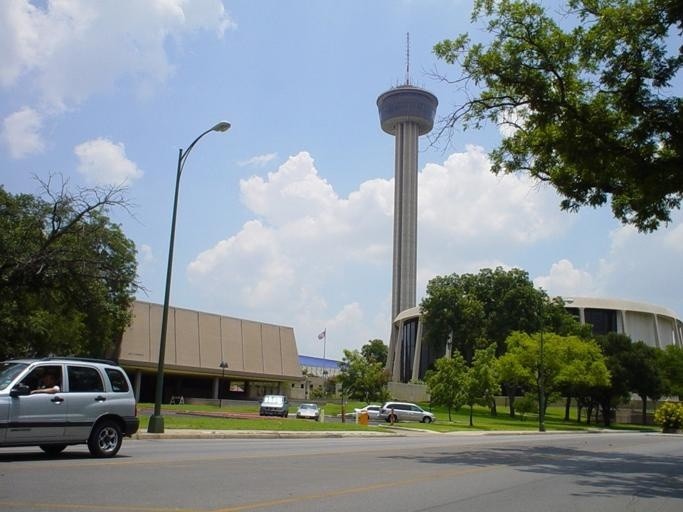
[217,361,228,408]
[537,298,574,432]
[148,121,233,433]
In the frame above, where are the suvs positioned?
[258,393,290,418]
[380,400,436,424]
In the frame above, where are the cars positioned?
[296,403,321,421]
[352,403,382,420]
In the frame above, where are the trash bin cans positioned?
[355,411,369,425]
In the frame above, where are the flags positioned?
[318,330,325,340]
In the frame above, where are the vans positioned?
[0,356,142,459]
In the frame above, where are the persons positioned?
[30,373,61,394]
